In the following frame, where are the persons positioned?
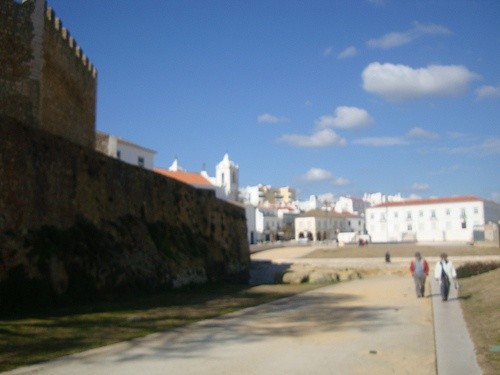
[410,251,429,298]
[358,239,369,246]
[435,252,457,301]
